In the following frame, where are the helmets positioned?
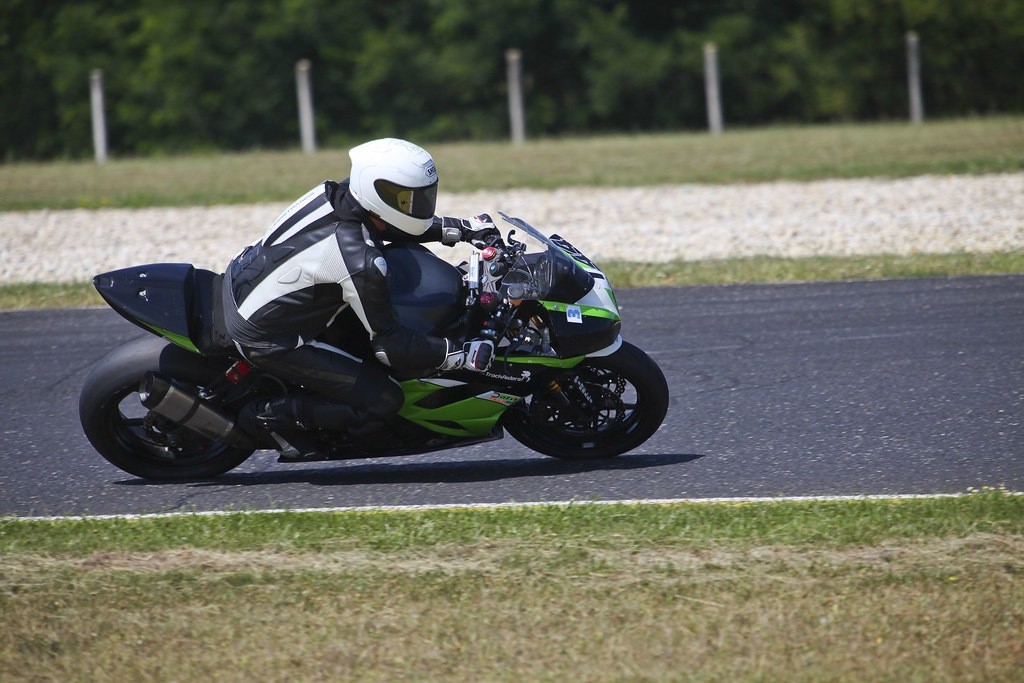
[348,138,439,236]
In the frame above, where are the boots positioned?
[239,386,323,460]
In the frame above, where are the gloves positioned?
[441,213,502,250]
[434,337,494,373]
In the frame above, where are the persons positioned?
[223,138,501,460]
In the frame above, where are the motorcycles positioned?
[77,209,670,483]
